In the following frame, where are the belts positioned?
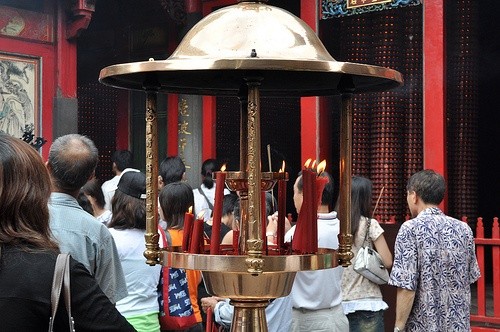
[293,307,314,314]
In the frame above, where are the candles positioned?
[277,160,285,252]
[210,165,227,255]
[292,157,329,254]
[192,210,208,252]
[261,191,267,255]
[181,206,194,251]
[233,225,240,253]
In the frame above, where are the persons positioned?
[1,129,482,332]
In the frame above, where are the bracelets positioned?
[393,327,405,332]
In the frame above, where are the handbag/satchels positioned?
[153,226,197,330]
[353,218,389,285]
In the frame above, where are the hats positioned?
[117,171,147,199]
